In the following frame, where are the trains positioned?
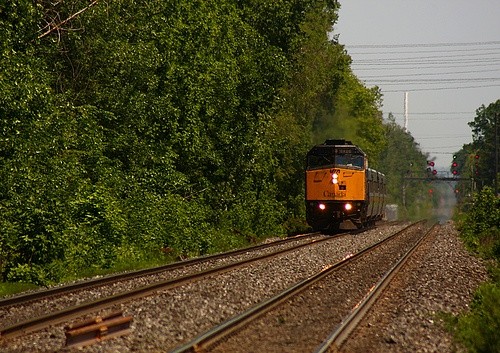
[306,137,387,235]
